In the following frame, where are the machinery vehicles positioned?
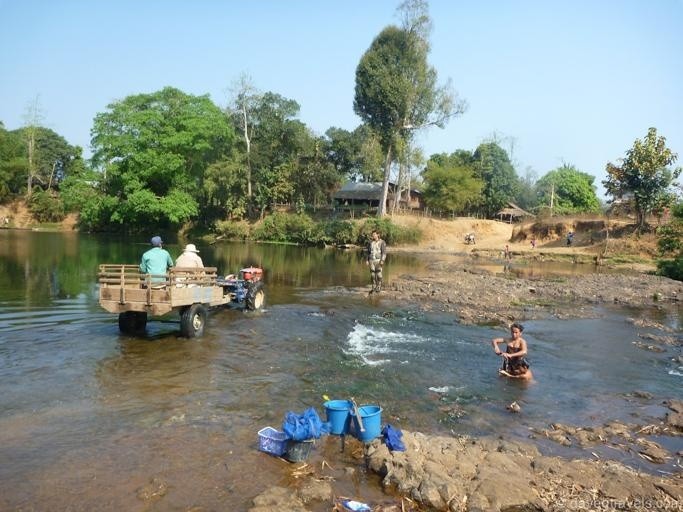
[99,263,266,337]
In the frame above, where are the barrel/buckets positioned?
[285,437,314,462]
[346,399,383,441]
[322,395,356,438]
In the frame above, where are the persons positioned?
[497,360,534,384]
[173,243,206,287]
[138,235,173,290]
[566,231,574,247]
[365,230,386,293]
[530,234,536,248]
[491,322,528,374]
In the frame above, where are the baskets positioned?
[257,426,289,456]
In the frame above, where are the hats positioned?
[151,236,163,244]
[182,244,200,253]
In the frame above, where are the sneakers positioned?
[373,284,381,292]
